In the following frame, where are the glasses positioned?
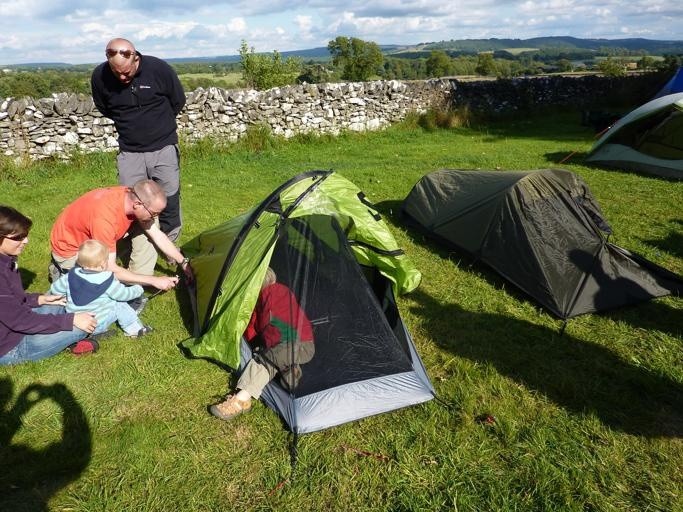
[105,48,138,60]
[2,231,28,241]
[142,204,160,218]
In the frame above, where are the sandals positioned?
[130,326,160,339]
[210,393,252,420]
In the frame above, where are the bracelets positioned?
[179,258,189,266]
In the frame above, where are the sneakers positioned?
[64,329,118,356]
[279,363,304,387]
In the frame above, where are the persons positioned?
[48,175,193,295]
[0,205,99,368]
[48,238,155,338]
[205,263,316,421]
[89,38,186,263]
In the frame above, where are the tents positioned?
[167,163,441,443]
[584,91,682,180]
[404,165,675,323]
[653,63,681,100]
